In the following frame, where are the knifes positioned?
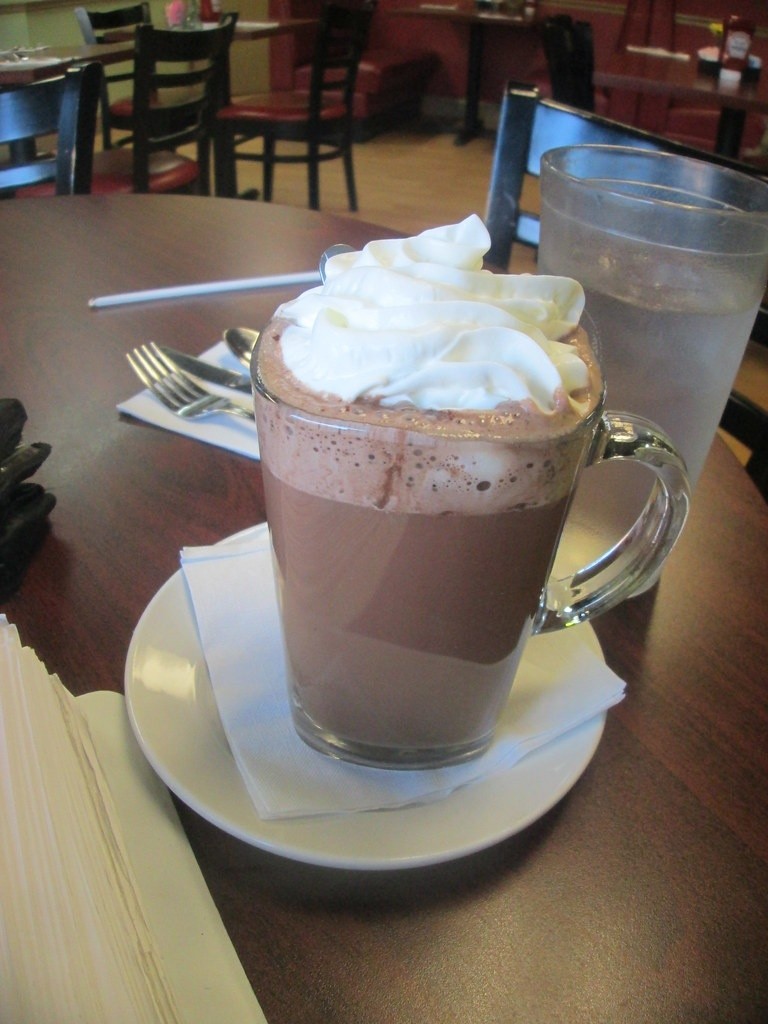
[156,346,252,394]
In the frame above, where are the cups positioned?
[249,315,693,771]
[535,143,768,487]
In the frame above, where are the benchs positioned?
[264,1,440,143]
[567,1,767,166]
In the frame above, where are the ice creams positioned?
[268,210,593,415]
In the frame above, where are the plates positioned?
[122,520,608,872]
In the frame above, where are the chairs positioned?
[544,15,595,113]
[216,0,377,211]
[0,62,101,192]
[73,1,212,146]
[483,81,767,501]
[100,11,240,194]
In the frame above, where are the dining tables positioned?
[0,194,766,1023]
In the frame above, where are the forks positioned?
[127,340,255,423]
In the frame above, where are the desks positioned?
[1,41,135,164]
[591,74,768,180]
[390,8,544,147]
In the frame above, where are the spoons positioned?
[224,327,259,368]
